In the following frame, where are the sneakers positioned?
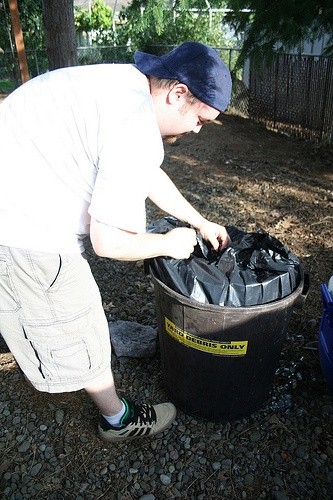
[98,398,177,442]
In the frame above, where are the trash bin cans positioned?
[142,216,311,425]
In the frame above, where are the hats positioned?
[134,41,232,114]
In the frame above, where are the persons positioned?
[1,41,232,444]
